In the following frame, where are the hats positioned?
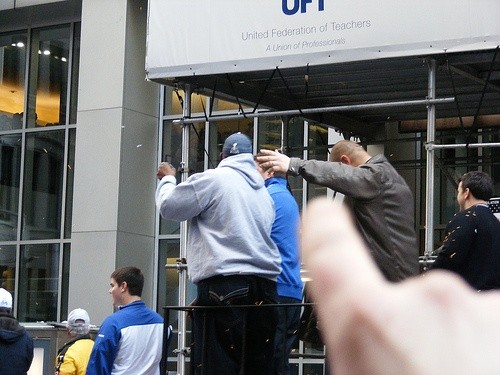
[223,132,252,154]
[67,308,90,327]
[0,288,12,308]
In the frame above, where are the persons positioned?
[254,151,303,375]
[298,198,500,375]
[154,132,282,375]
[431,171,500,292]
[84,266,174,375]
[0,287,34,375]
[54,308,95,375]
[256,140,420,281]
